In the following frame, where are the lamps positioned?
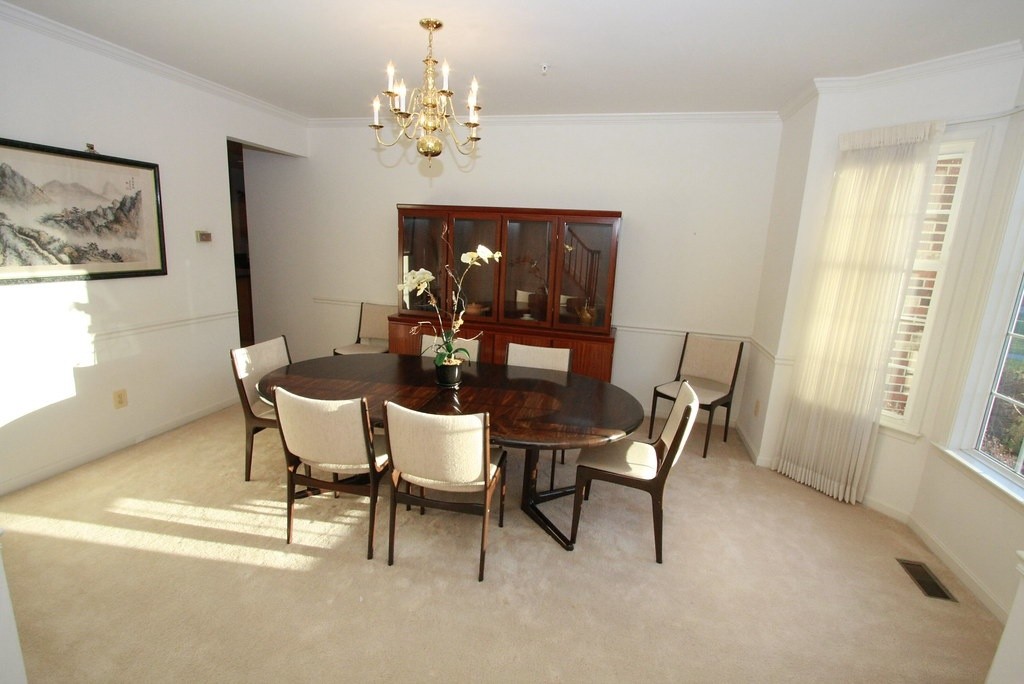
[369,18,482,166]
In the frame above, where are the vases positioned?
[433,359,462,389]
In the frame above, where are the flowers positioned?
[396,244,503,365]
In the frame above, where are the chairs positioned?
[229,335,312,490]
[419,335,481,362]
[380,399,507,582]
[272,385,412,559]
[648,333,743,457]
[333,302,399,356]
[569,379,699,564]
[499,342,572,490]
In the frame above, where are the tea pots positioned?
[574,296,597,326]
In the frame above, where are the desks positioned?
[258,354,644,551]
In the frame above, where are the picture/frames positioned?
[0,138,168,285]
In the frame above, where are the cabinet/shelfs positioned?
[388,204,622,383]
